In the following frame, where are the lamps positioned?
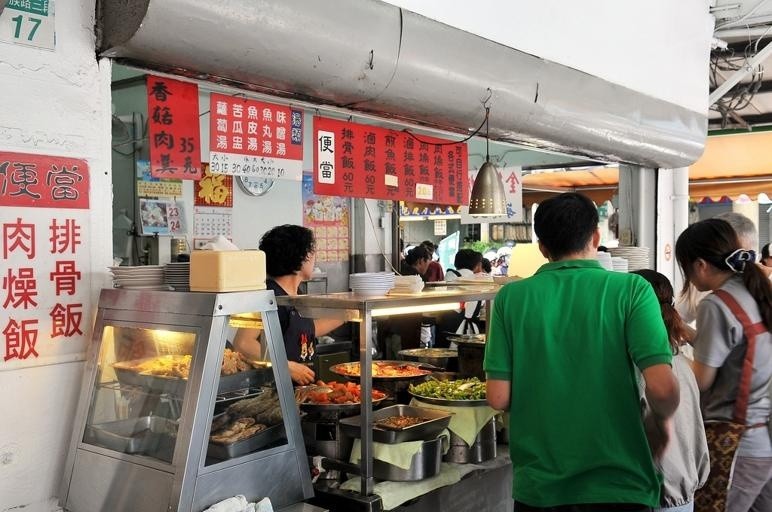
[469,109,507,216]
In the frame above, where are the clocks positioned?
[236,176,277,197]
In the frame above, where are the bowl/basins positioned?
[396,348,461,372]
[293,382,388,418]
[328,360,435,383]
[407,381,490,407]
[445,333,485,347]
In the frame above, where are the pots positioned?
[441,409,498,464]
[372,434,447,482]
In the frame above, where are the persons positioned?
[232,224,357,392]
[375,239,498,363]
[674,219,772,511]
[671,212,772,326]
[625,267,715,511]
[480,194,683,511]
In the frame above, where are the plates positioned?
[596,246,651,273]
[107,261,190,292]
[348,272,396,296]
